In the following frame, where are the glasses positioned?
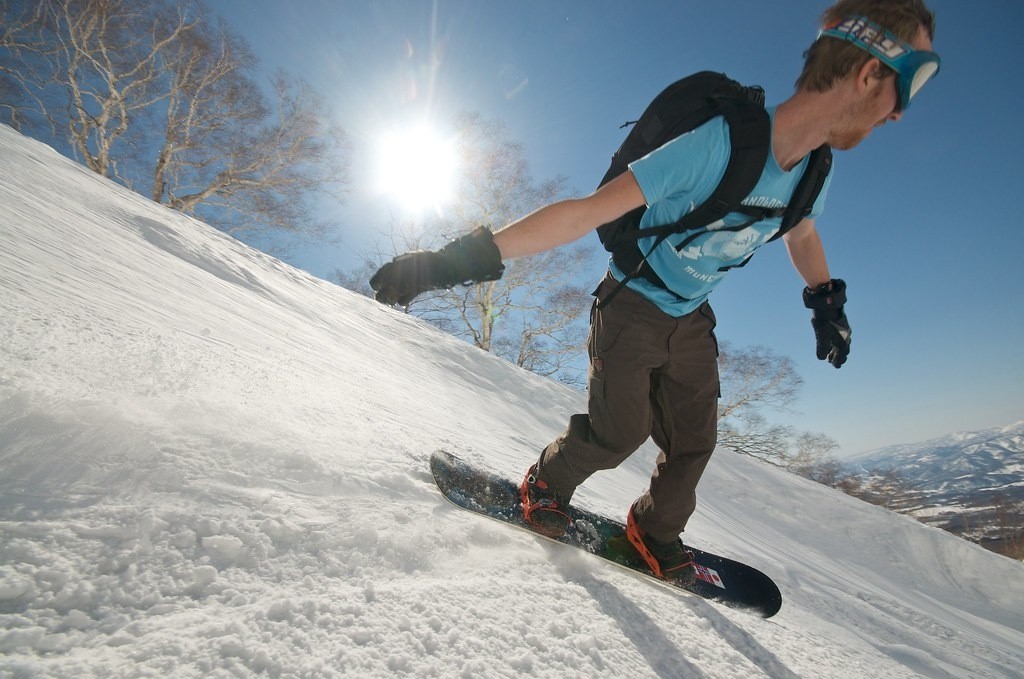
[831,15,943,114]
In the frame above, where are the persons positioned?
[367,0,941,586]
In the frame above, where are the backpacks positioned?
[592,70,834,288]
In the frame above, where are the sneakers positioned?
[519,459,572,537]
[627,499,697,585]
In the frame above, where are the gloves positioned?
[369,225,505,306]
[803,277,853,369]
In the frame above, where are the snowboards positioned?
[431,447,783,621]
